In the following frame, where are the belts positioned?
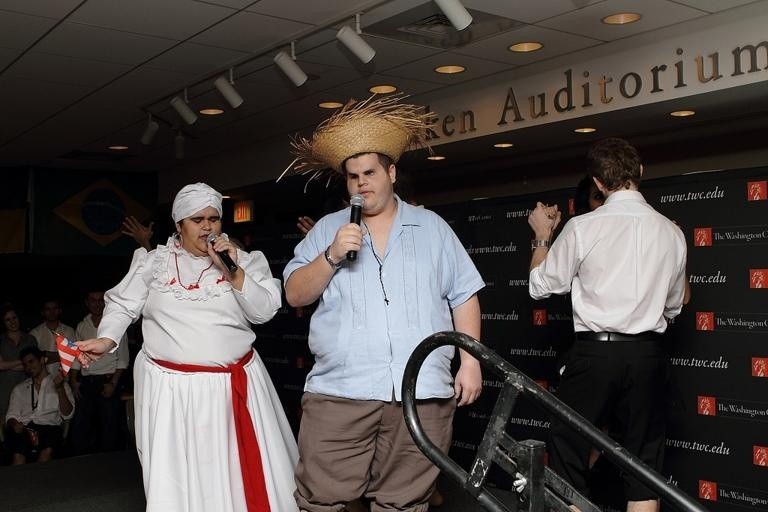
[578,332,636,343]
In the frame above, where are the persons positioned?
[0,215,155,465]
[74,182,300,512]
[541,176,692,512]
[527,138,688,512]
[282,153,487,512]
[296,188,444,512]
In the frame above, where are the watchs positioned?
[530,240,551,253]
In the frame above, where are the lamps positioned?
[137,0,473,150]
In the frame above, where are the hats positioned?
[276,91,439,194]
[171,181,224,224]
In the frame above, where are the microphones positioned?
[346,194,366,261]
[207,232,238,272]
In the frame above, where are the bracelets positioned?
[325,245,342,269]
